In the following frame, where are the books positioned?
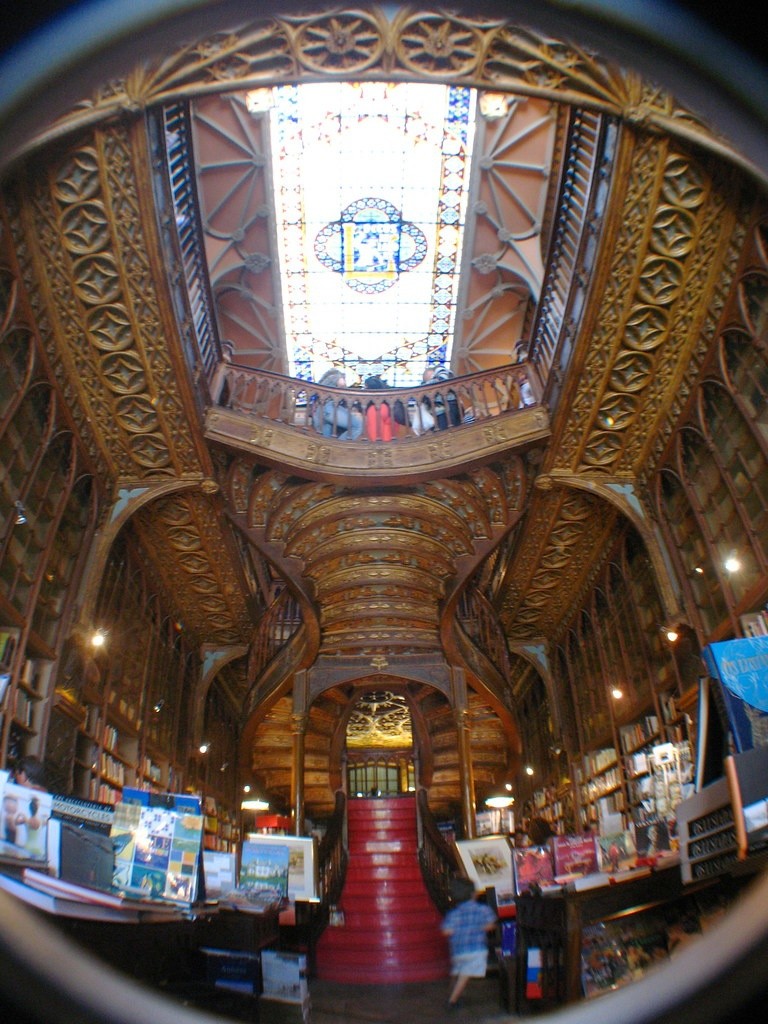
[474,698,693,898]
[0,627,288,913]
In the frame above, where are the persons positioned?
[441,878,497,1007]
[527,818,557,845]
[311,369,361,441]
[422,366,461,432]
[609,842,620,873]
[519,373,536,407]
[16,797,47,856]
[7,755,48,792]
[364,377,399,441]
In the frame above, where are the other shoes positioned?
[445,1000,460,1009]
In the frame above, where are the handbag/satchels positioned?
[394,399,412,427]
[412,403,435,436]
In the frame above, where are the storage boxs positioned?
[261,948,309,1000]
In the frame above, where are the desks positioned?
[483,860,683,1013]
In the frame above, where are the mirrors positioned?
[0,0,768,1024]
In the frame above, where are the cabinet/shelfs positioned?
[50,902,328,1024]
[0,267,245,855]
[511,268,768,846]
